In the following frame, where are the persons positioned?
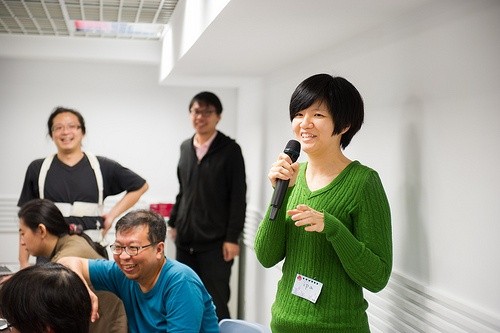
[166,91,247,324]
[253,74,393,333]
[0,200,221,333]
[15,107,149,269]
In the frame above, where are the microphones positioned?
[269,140,301,221]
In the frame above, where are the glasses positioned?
[51,122,81,133]
[191,108,215,117]
[110,243,153,256]
